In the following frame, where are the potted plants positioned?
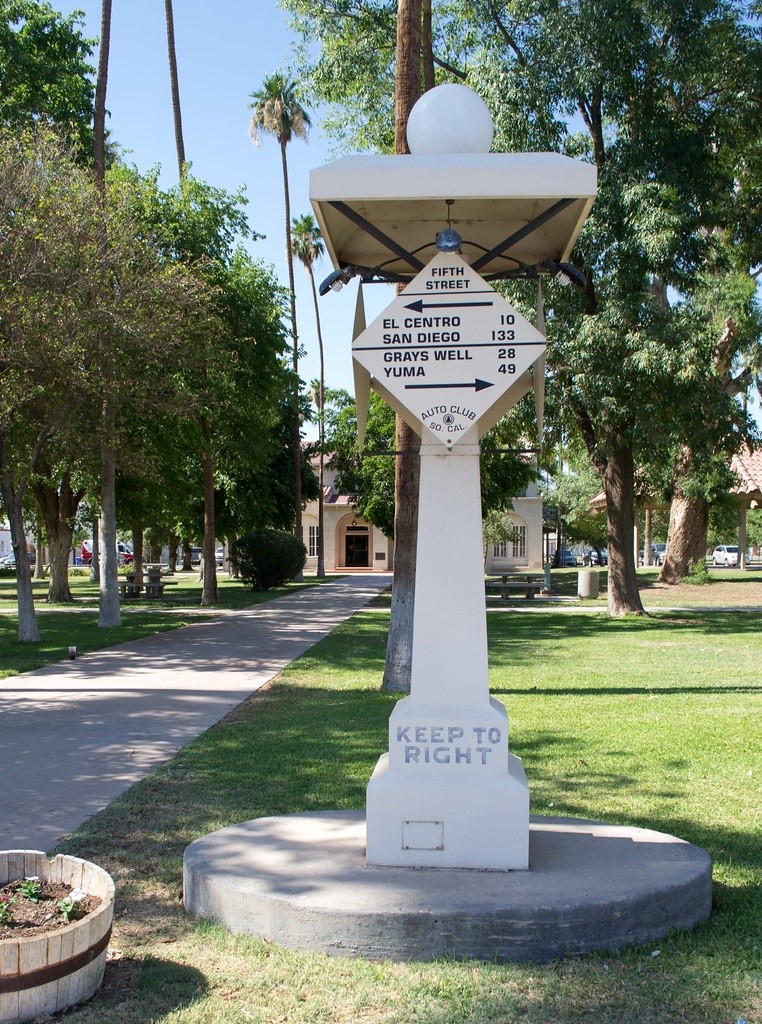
[0,849,116,1023]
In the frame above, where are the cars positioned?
[215,547,224,564]
[638,544,666,566]
[0,552,44,566]
[583,550,608,567]
[550,550,577,567]
[712,545,750,567]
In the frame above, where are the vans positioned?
[81,540,133,565]
[180,547,202,565]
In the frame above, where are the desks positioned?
[487,572,546,600]
[118,573,174,598]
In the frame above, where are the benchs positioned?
[117,582,178,587]
[485,582,547,587]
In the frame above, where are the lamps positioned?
[320,265,357,296]
[405,84,495,154]
[538,257,588,288]
[352,520,357,526]
[436,199,462,256]
